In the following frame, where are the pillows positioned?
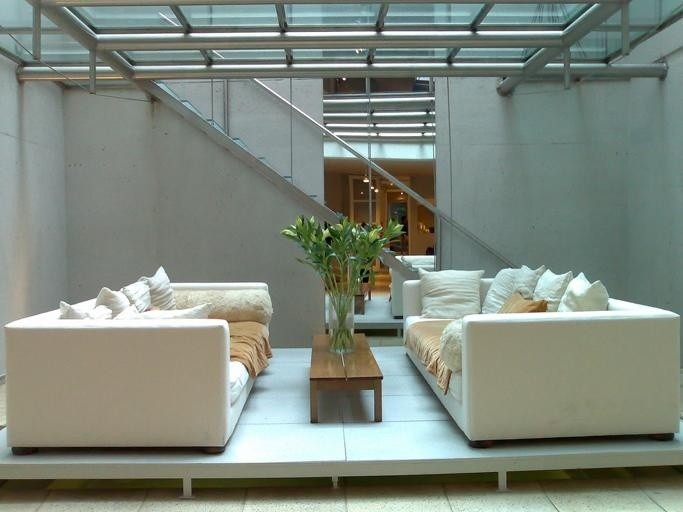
[58,266,274,321]
[417,264,609,319]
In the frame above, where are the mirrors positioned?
[323,77,436,321]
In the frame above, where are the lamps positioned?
[363,167,370,183]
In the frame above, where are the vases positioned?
[328,295,354,354]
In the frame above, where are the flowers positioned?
[279,213,407,354]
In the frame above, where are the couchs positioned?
[5,281,271,455]
[402,277,681,449]
[388,255,435,319]
